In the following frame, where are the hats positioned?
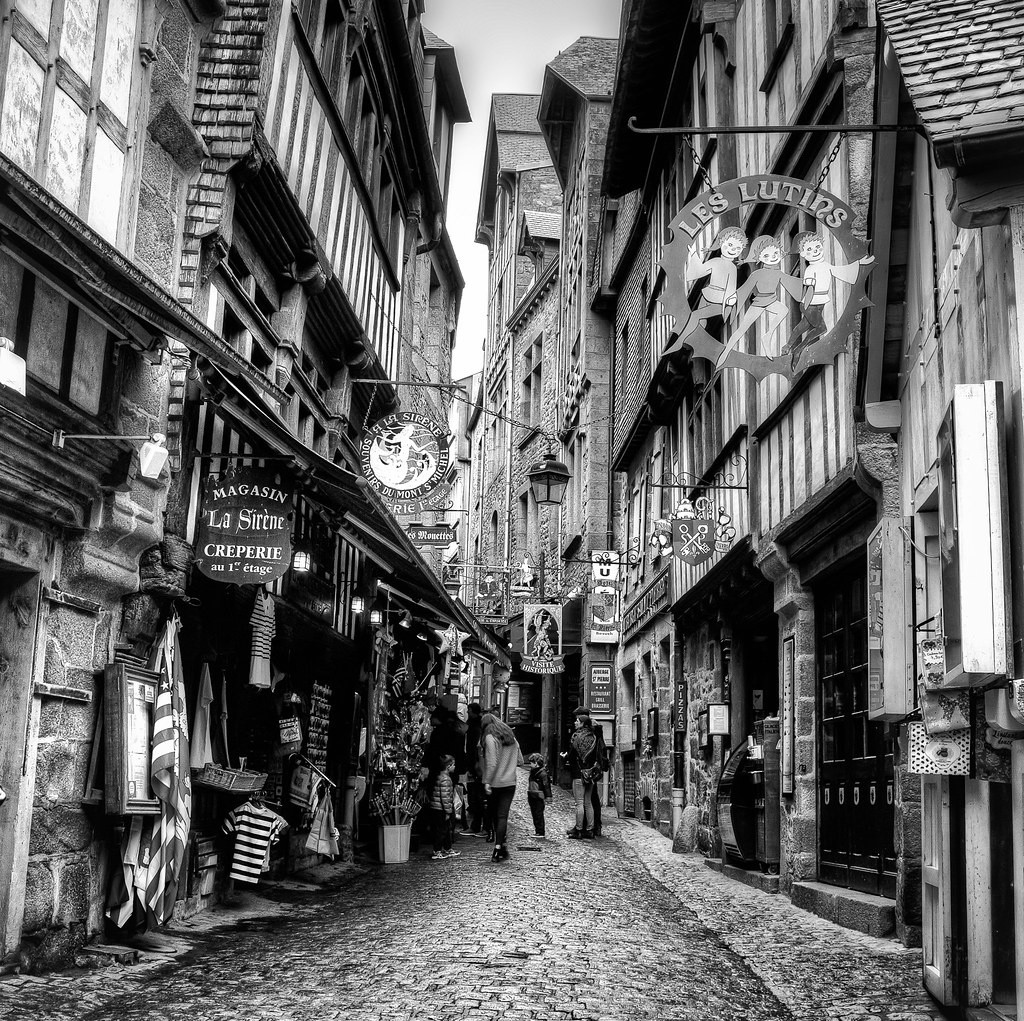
[428,706,450,718]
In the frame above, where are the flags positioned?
[104,618,193,929]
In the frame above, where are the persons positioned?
[479,714,524,862]
[430,755,462,859]
[560,706,607,840]
[430,703,488,837]
[527,753,553,838]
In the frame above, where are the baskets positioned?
[200,763,268,792]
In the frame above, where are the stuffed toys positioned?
[492,663,512,693]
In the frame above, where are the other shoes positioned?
[582,830,595,839]
[459,829,475,835]
[475,829,488,837]
[498,844,510,862]
[568,831,582,839]
[491,848,501,863]
[486,830,494,843]
[593,829,602,836]
[531,833,545,838]
[566,826,578,834]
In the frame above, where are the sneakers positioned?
[431,850,449,859]
[445,849,461,856]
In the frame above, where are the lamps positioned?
[383,609,413,629]
[338,580,365,614]
[0,337,27,398]
[527,435,573,506]
[52,428,169,479]
[412,621,428,641]
[290,532,315,572]
[367,595,384,624]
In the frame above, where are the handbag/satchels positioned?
[581,762,604,786]
